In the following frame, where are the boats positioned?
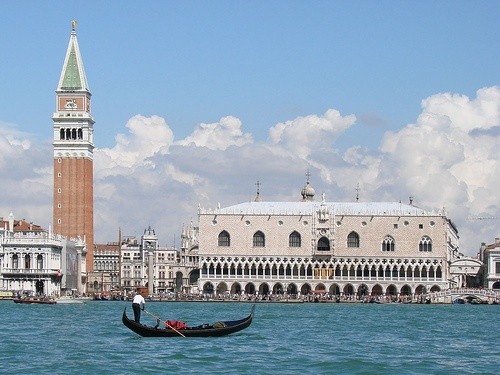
[0,291,17,300]
[56,290,94,304]
[122,303,256,337]
[13,291,57,304]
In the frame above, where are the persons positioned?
[131,291,146,325]
[73,287,499,303]
[12,288,54,303]
